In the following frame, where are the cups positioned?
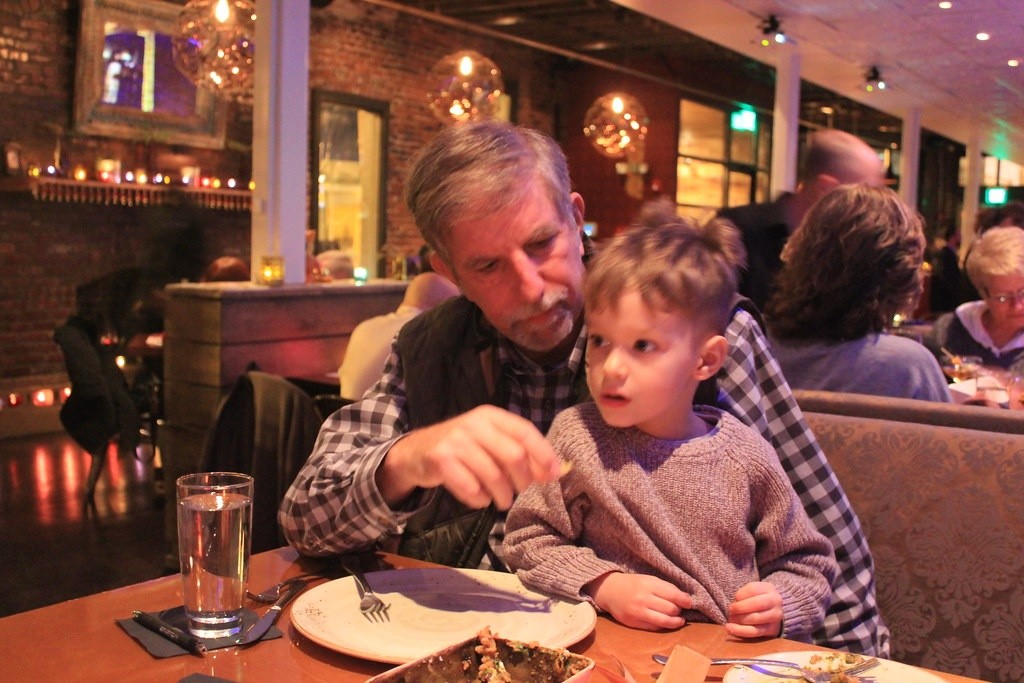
[176,471,254,639]
[942,355,983,383]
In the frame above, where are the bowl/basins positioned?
[365,636,595,683]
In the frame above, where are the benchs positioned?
[791,388,1024,683]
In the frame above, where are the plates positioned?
[722,650,945,683]
[289,567,598,665]
[946,377,1008,404]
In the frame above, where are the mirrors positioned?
[310,90,390,278]
[71,0,229,150]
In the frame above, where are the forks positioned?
[651,650,882,683]
[247,572,326,601]
[338,553,392,614]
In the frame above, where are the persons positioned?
[340,271,465,398]
[759,183,952,402]
[716,128,884,317]
[485,196,838,643]
[57,248,354,457]
[277,120,891,660]
[898,225,1024,410]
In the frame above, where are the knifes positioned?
[235,579,307,645]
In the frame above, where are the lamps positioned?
[422,0,504,125]
[582,6,650,162]
[172,0,257,104]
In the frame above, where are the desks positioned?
[0,545,992,683]
